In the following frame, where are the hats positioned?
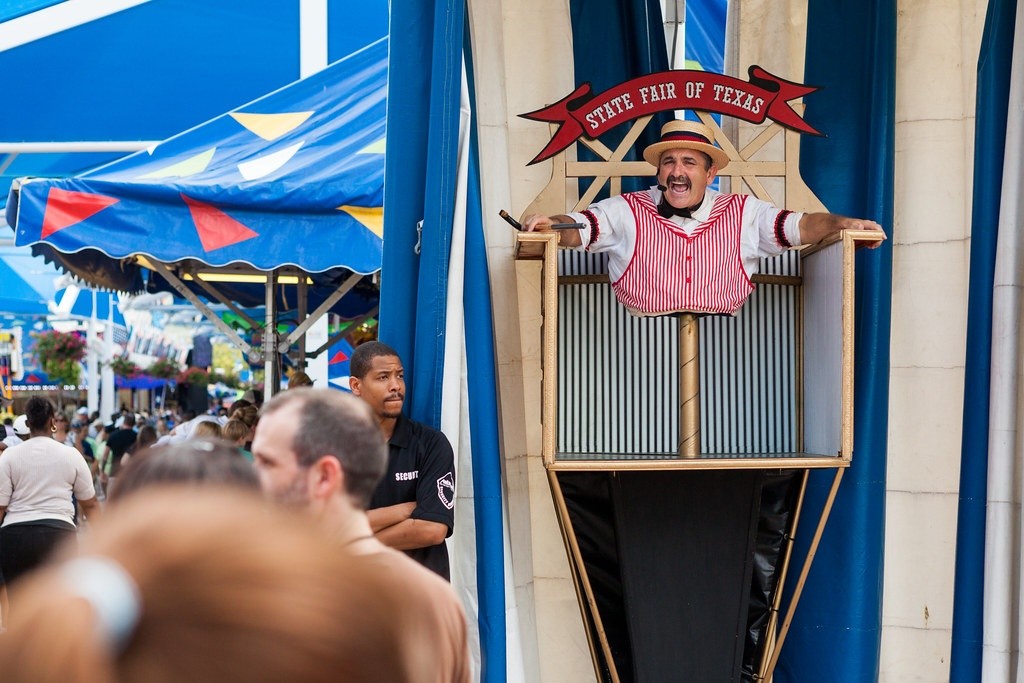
[643,119,729,170]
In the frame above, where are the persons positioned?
[522,120,886,314]
[0,318,474,683]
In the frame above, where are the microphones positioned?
[656,168,667,192]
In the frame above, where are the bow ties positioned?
[657,193,705,219]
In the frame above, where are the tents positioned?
[9,40,394,410]
